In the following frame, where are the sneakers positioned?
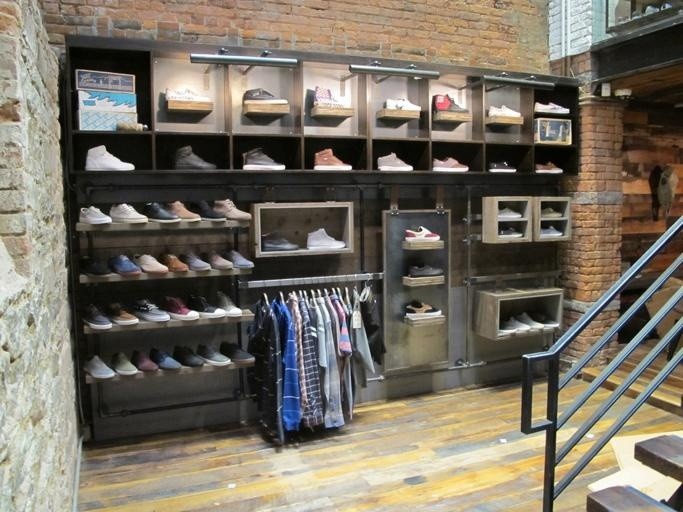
[165,88,213,101]
[214,289,242,317]
[516,311,544,329]
[82,355,115,379]
[172,144,216,170]
[243,88,288,105]
[108,254,141,275]
[432,94,469,113]
[144,202,181,223]
[132,296,171,322]
[498,207,522,219]
[82,303,112,330]
[488,104,521,118]
[498,226,523,237]
[221,249,254,269]
[132,254,168,274]
[79,206,112,225]
[314,148,352,170]
[408,263,444,277]
[191,200,227,222]
[109,203,148,224]
[502,316,530,331]
[85,144,135,171]
[540,225,563,238]
[535,161,563,173]
[201,250,233,270]
[306,228,346,250]
[106,301,139,325]
[173,345,203,367]
[158,251,188,272]
[405,302,442,318]
[188,296,226,319]
[313,86,345,109]
[219,342,255,364]
[532,311,560,327]
[80,256,111,278]
[405,225,440,242]
[432,157,469,172]
[178,252,211,271]
[213,199,252,220]
[241,147,285,170]
[263,237,299,251]
[105,352,137,375]
[196,343,231,367]
[489,160,517,173]
[159,293,199,321]
[149,346,182,370]
[385,97,421,111]
[533,101,570,114]
[542,206,562,218]
[131,350,158,373]
[377,152,413,171]
[167,201,201,222]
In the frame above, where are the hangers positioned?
[257,273,372,314]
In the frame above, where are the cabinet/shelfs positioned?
[251,202,355,258]
[382,208,451,373]
[70,183,258,441]
[474,287,564,340]
[66,35,579,178]
[481,194,532,244]
[535,195,573,243]
